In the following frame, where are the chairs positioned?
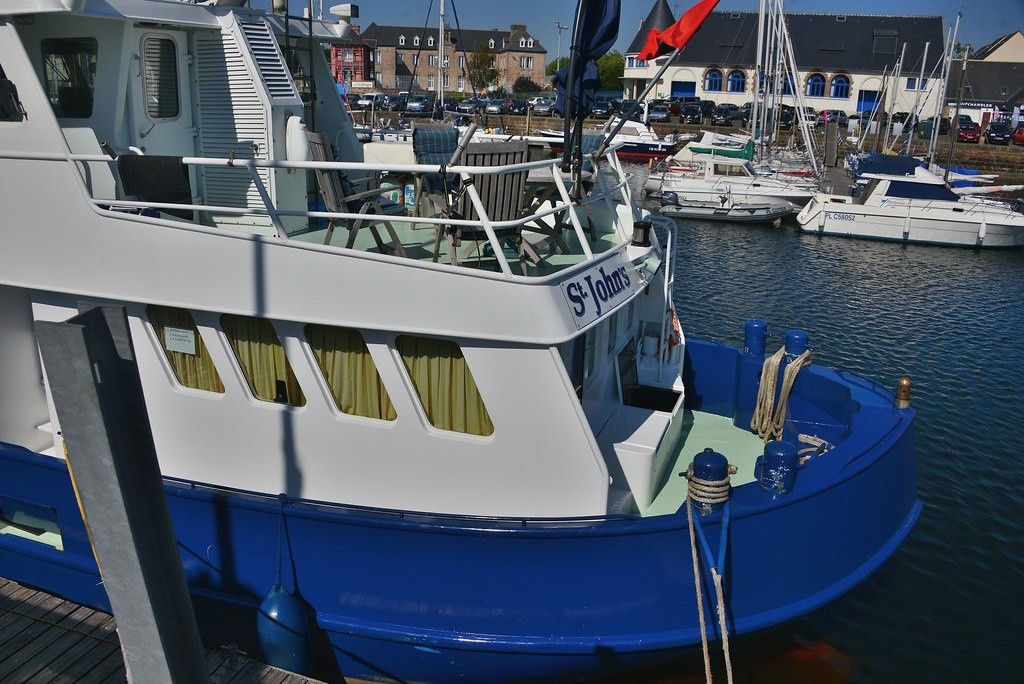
[410,121,460,244]
[429,139,529,279]
[305,129,408,260]
[523,129,608,242]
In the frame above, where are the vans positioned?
[356,92,385,109]
[661,96,700,116]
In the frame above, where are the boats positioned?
[0,0,927,684]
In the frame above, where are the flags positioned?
[1011,106,1020,131]
[633,0,721,60]
[993,105,999,120]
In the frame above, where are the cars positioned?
[797,113,820,133]
[817,109,848,127]
[456,97,481,114]
[955,123,980,143]
[509,99,532,116]
[526,96,544,111]
[985,121,1013,146]
[1012,124,1024,146]
[648,105,671,122]
[863,109,888,124]
[532,98,556,117]
[436,98,458,114]
[679,105,703,124]
[485,99,510,115]
[712,103,742,120]
[710,107,733,126]
[589,100,614,120]
[342,95,362,111]
[502,97,519,109]
[886,111,919,132]
[742,110,761,129]
[381,95,406,111]
[846,114,880,131]
[928,116,950,135]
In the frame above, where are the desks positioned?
[458,165,593,269]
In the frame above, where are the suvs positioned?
[354,0,1024,252]
[951,114,972,129]
[618,99,641,122]
[405,94,435,113]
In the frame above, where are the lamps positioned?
[330,3,359,26]
[633,256,662,277]
